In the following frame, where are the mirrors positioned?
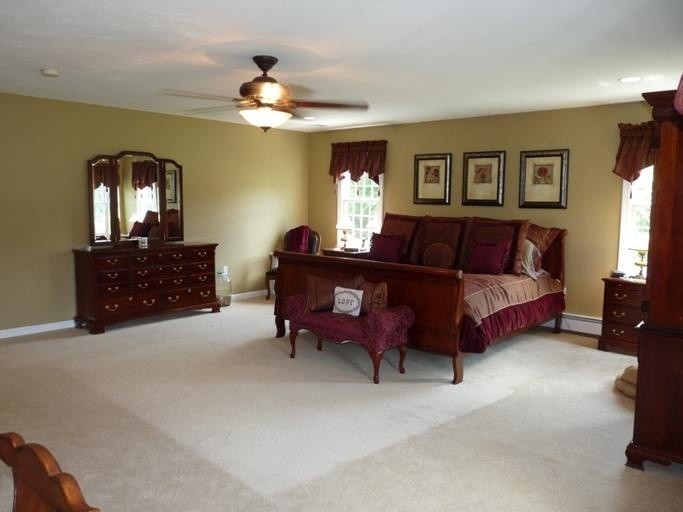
[88,151,183,246]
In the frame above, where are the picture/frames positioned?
[166,170,176,203]
[414,153,451,204]
[462,151,505,206]
[520,149,569,208]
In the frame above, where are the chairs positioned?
[0,432,101,512]
[266,227,320,300]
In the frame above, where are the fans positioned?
[163,56,368,120]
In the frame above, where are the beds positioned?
[274,229,569,384]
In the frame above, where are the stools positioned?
[287,294,414,383]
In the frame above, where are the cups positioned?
[138,236,148,249]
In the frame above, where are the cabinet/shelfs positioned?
[625,90,683,471]
[72,243,221,334]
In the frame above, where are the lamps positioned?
[239,107,293,132]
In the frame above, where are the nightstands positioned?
[320,248,370,259]
[599,277,646,351]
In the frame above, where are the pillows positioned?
[305,275,365,312]
[370,212,561,274]
[146,225,159,237]
[332,286,364,316]
[288,226,309,253]
[167,209,177,233]
[358,280,388,314]
[143,211,158,224]
[129,221,151,237]
